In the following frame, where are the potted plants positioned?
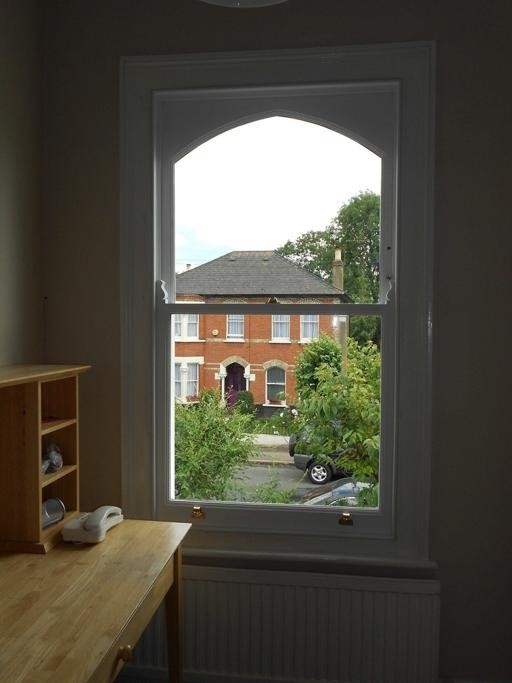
[276,391,289,405]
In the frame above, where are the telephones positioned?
[62,506,122,543]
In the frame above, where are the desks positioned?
[0,511,192,683]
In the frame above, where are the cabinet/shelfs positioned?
[0,362,92,554]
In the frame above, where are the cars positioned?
[286,476,375,507]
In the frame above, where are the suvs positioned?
[291,416,356,485]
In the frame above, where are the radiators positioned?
[120,565,443,683]
